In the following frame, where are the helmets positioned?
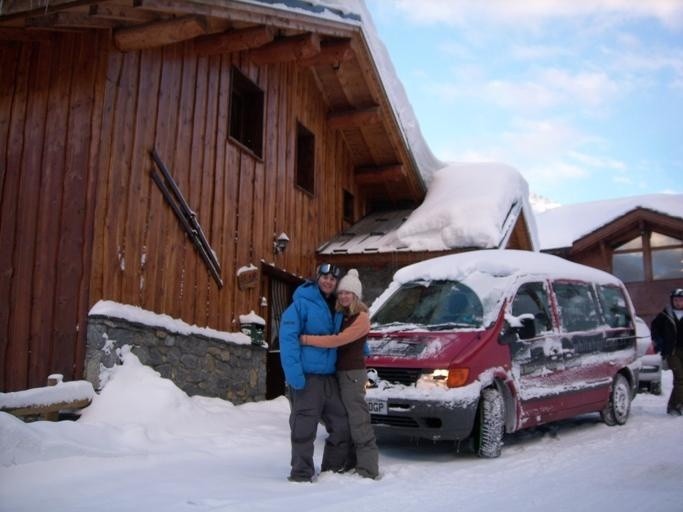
[670,289,683,297]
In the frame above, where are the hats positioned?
[337,268,363,298]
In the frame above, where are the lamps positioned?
[272,232,289,254]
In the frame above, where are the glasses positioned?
[319,263,342,278]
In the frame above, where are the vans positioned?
[364,273,640,457]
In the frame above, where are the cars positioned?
[636,315,662,395]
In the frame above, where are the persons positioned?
[297,266,382,481]
[650,286,683,415]
[276,260,356,480]
